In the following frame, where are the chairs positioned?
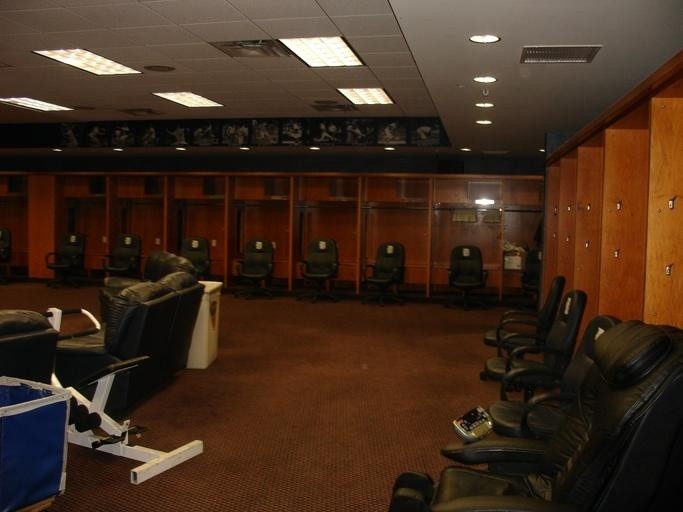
[445,243,491,303]
[391,276,682,511]
[232,237,276,302]
[295,236,342,306]
[46,234,87,290]
[0,273,204,458]
[102,235,144,273]
[360,239,409,308]
[176,236,212,275]
